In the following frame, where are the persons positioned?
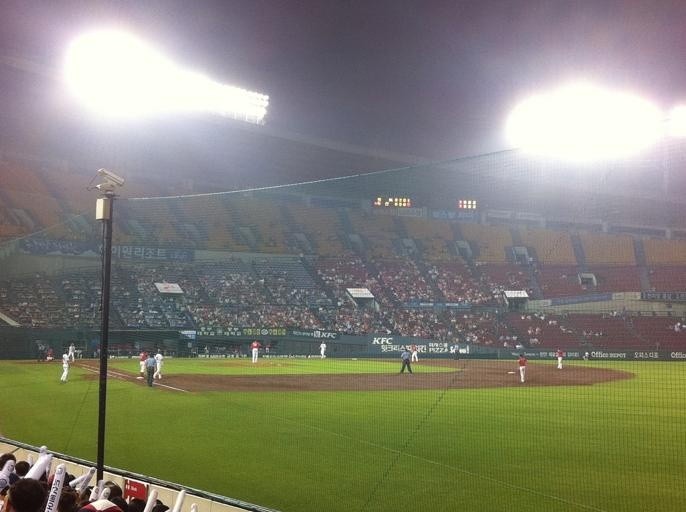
[60,350,72,383]
[556,347,563,370]
[0,453,170,511]
[399,349,413,374]
[517,354,527,383]
[137,350,149,379]
[152,351,164,380]
[668,319,686,330]
[143,353,157,387]
